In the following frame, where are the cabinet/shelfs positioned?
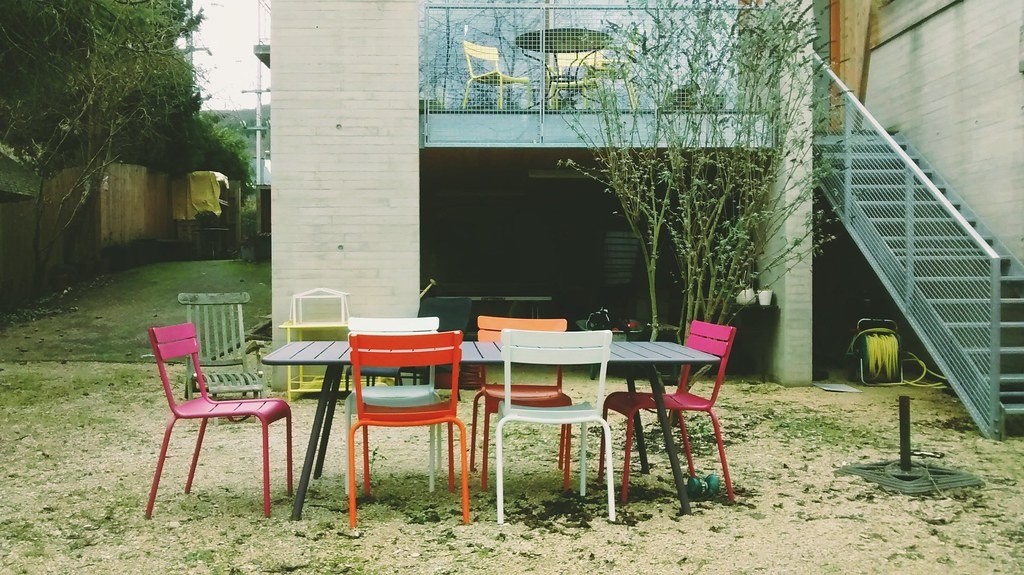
[279,287,398,400]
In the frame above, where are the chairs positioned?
[144,322,293,518]
[348,330,469,528]
[595,28,640,110]
[463,40,531,111]
[176,290,265,427]
[346,297,473,397]
[470,315,571,492]
[344,317,439,496]
[599,320,738,506]
[548,45,602,109]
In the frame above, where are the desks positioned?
[260,340,720,521]
